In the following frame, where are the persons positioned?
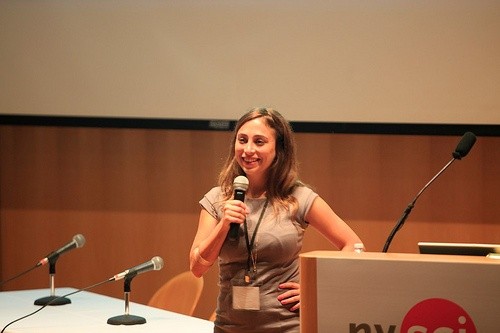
[190,107,366,333]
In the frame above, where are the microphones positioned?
[228,176,249,241]
[37,234,86,267]
[383,132,477,253]
[109,256,164,282]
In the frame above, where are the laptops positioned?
[418,242,500,257]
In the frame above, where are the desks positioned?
[0,288,216,333]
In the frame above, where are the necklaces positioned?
[244,193,270,274]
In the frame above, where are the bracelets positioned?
[193,245,213,267]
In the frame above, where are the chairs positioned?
[146,269,205,316]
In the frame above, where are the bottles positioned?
[354,243,364,252]
